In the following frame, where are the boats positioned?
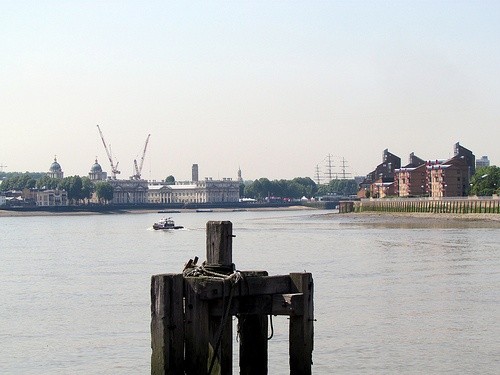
[153,217,184,229]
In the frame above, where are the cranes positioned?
[97,125,121,180]
[133,133,151,179]
